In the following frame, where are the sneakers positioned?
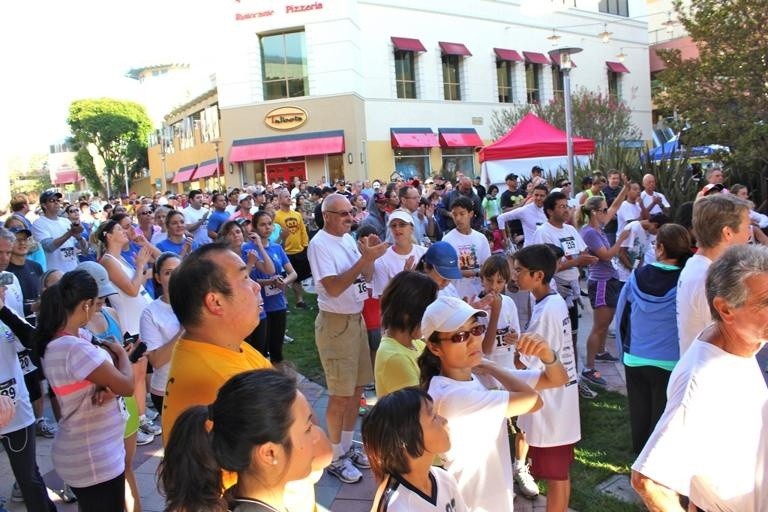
[140,422,162,436]
[607,330,618,339]
[296,302,306,307]
[344,447,371,470]
[326,455,364,485]
[12,481,24,503]
[63,482,79,503]
[512,466,539,498]
[136,432,154,446]
[145,409,160,421]
[283,335,294,344]
[595,351,620,364]
[581,368,609,387]
[579,382,599,401]
[580,287,589,298]
[35,418,55,438]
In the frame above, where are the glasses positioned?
[510,177,518,181]
[412,196,422,200]
[138,210,154,217]
[285,183,289,186]
[336,184,345,188]
[279,183,284,185]
[69,208,80,213]
[49,198,61,203]
[327,209,353,217]
[510,266,539,277]
[438,322,486,345]
[390,222,411,228]
[231,191,239,195]
[15,237,29,243]
[595,207,608,216]
[561,181,572,187]
[554,203,569,209]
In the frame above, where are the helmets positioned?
[109,195,117,200]
[121,193,129,199]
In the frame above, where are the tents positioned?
[478,111,596,195]
[642,137,716,193]
[650,128,730,186]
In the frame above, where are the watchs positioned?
[540,349,560,367]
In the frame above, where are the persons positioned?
[389,171,405,214]
[416,295,568,512]
[731,186,767,246]
[489,216,502,252]
[386,186,423,245]
[136,189,190,211]
[577,176,607,230]
[578,171,631,386]
[1,297,59,512]
[374,271,440,399]
[530,192,600,400]
[225,186,240,216]
[639,174,671,221]
[3,227,56,439]
[88,220,162,445]
[109,212,163,423]
[159,240,333,512]
[415,240,459,300]
[208,194,232,241]
[242,211,296,367]
[373,210,429,297]
[70,261,146,512]
[10,194,34,239]
[266,181,279,218]
[696,166,728,200]
[4,216,47,273]
[616,182,649,247]
[501,173,526,250]
[482,185,499,238]
[412,175,452,247]
[613,223,694,459]
[306,195,388,483]
[291,176,308,223]
[246,178,266,216]
[235,218,251,241]
[471,254,540,497]
[323,175,350,199]
[362,193,388,245]
[385,191,400,215]
[42,267,65,290]
[629,243,767,512]
[141,253,185,415]
[0,226,65,502]
[610,212,667,314]
[37,269,133,512]
[111,190,138,229]
[441,197,491,308]
[602,168,627,251]
[272,190,309,310]
[227,194,254,223]
[412,196,435,246]
[350,196,367,223]
[31,190,88,276]
[315,187,334,231]
[81,193,111,231]
[520,165,548,205]
[355,226,381,415]
[179,191,214,254]
[438,177,485,235]
[222,222,276,357]
[152,208,174,247]
[497,185,549,250]
[677,193,753,362]
[65,204,98,263]
[156,211,198,260]
[361,387,469,511]
[556,179,577,224]
[149,209,186,247]
[513,244,582,512]
[350,178,389,242]
[305,188,322,277]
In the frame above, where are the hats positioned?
[419,294,492,347]
[385,211,414,230]
[375,193,389,202]
[168,194,176,199]
[237,193,253,203]
[75,261,120,299]
[575,192,585,209]
[424,178,435,184]
[130,191,137,196]
[336,180,345,183]
[10,225,35,240]
[426,241,464,283]
[40,191,62,204]
[550,188,567,195]
[154,191,162,196]
[403,180,421,188]
[175,194,183,199]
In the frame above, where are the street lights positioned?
[121,158,130,197]
[209,138,224,189]
[211,137,223,194]
[160,156,166,192]
